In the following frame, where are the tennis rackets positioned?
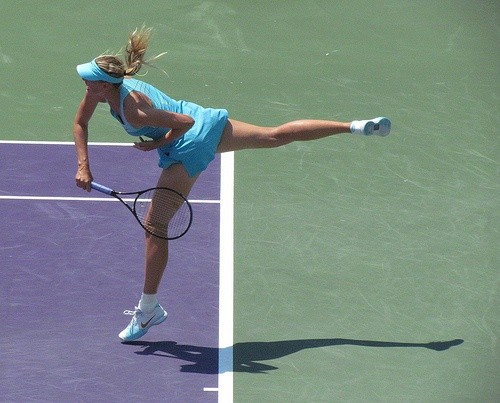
[91,180,194,240]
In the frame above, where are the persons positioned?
[74,21,392,344]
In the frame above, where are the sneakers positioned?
[118,303,167,342]
[350,117,392,137]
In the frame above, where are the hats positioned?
[76,56,125,83]
